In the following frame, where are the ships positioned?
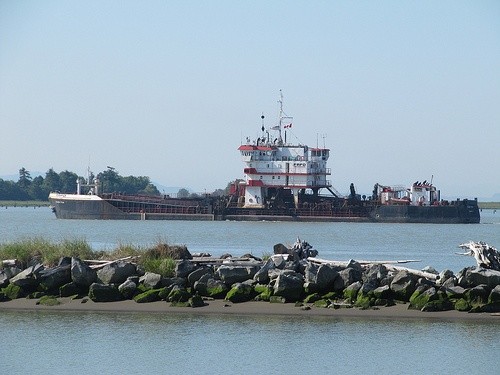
[47,88,480,225]
